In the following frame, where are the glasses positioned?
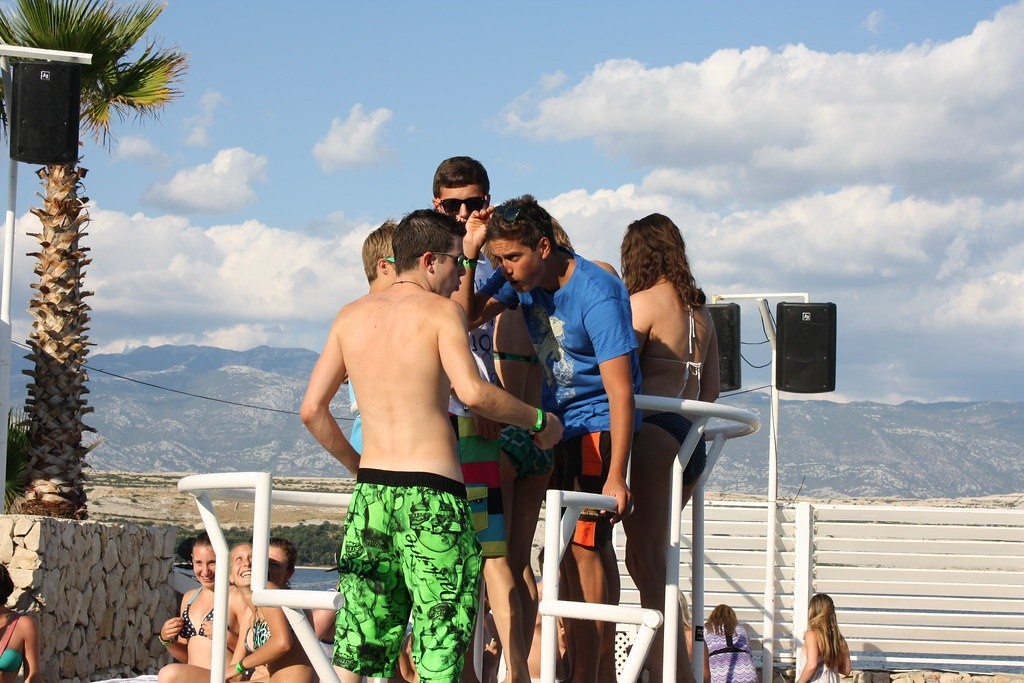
[431,252,465,267]
[436,195,487,215]
[493,205,546,237]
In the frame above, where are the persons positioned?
[296,154,644,682]
[794,592,852,682]
[0,562,46,683]
[614,209,723,682]
[674,588,760,681]
[152,527,339,681]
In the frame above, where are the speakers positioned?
[9,61,81,166]
[706,303,740,393]
[775,303,837,394]
